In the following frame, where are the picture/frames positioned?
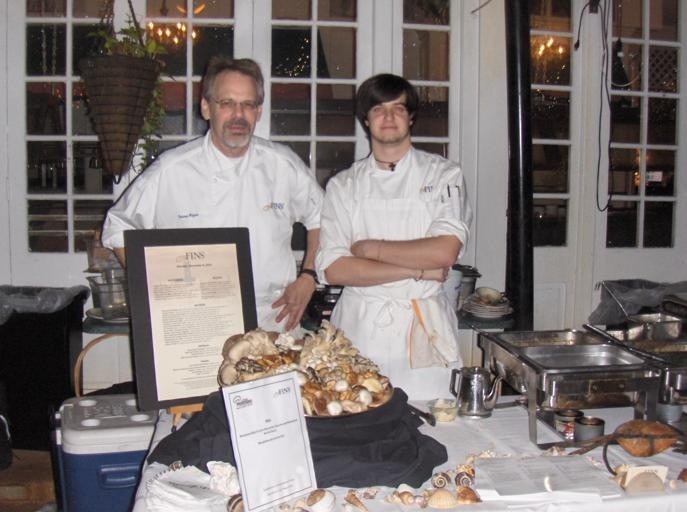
[123,227,258,412]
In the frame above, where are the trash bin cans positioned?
[590,279,687,330]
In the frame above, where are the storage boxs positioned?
[47,394,158,512]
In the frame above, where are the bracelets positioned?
[296,267,320,288]
[413,268,427,282]
[376,238,384,261]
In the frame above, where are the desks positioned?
[131,395,687,512]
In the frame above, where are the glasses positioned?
[208,94,258,109]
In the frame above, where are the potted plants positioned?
[78,1,169,184]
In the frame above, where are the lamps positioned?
[148,17,198,47]
[528,0,571,60]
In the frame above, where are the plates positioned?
[463,296,513,321]
[86,307,130,324]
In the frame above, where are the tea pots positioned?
[449,366,503,419]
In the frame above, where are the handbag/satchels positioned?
[147,389,448,487]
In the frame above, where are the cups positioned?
[443,271,462,313]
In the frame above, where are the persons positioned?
[98,53,324,342]
[314,72,472,400]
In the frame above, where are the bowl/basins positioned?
[476,286,502,303]
[427,399,458,421]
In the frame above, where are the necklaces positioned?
[374,154,401,171]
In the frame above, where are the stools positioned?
[74,317,132,397]
